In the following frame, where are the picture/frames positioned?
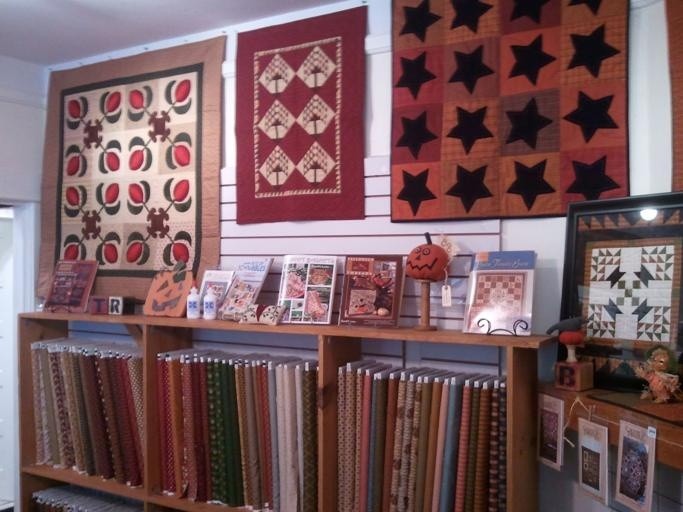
[556,191,683,396]
[42,258,99,313]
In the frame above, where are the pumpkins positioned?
[405,233,450,281]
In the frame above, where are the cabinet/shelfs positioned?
[13,310,559,512]
